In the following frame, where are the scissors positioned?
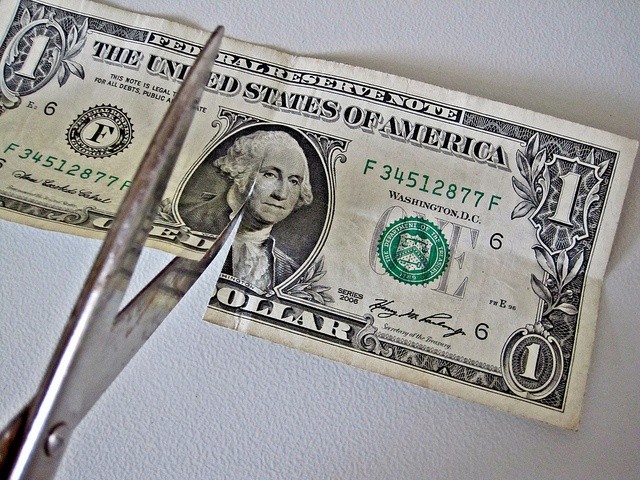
[0,24,241,480]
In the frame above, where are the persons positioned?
[182,131,313,292]
